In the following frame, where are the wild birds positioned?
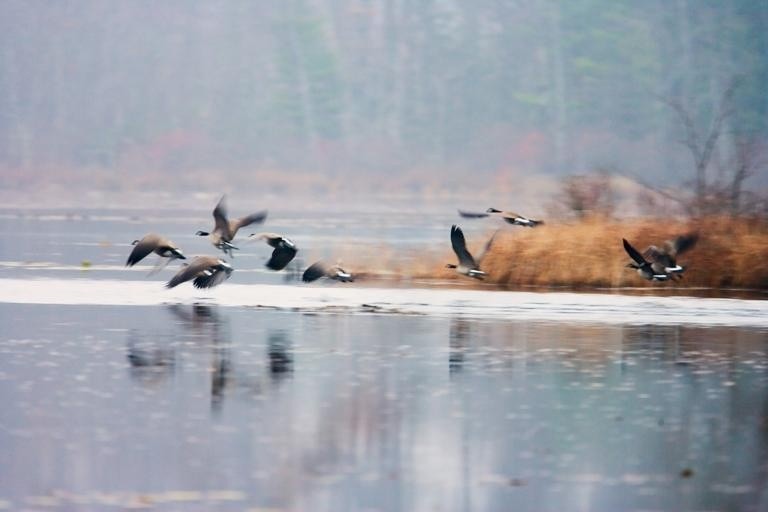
[457,206,543,229]
[124,231,185,279]
[301,259,355,283]
[243,231,299,272]
[444,224,499,281]
[165,250,234,291]
[620,227,699,288]
[196,193,268,261]
